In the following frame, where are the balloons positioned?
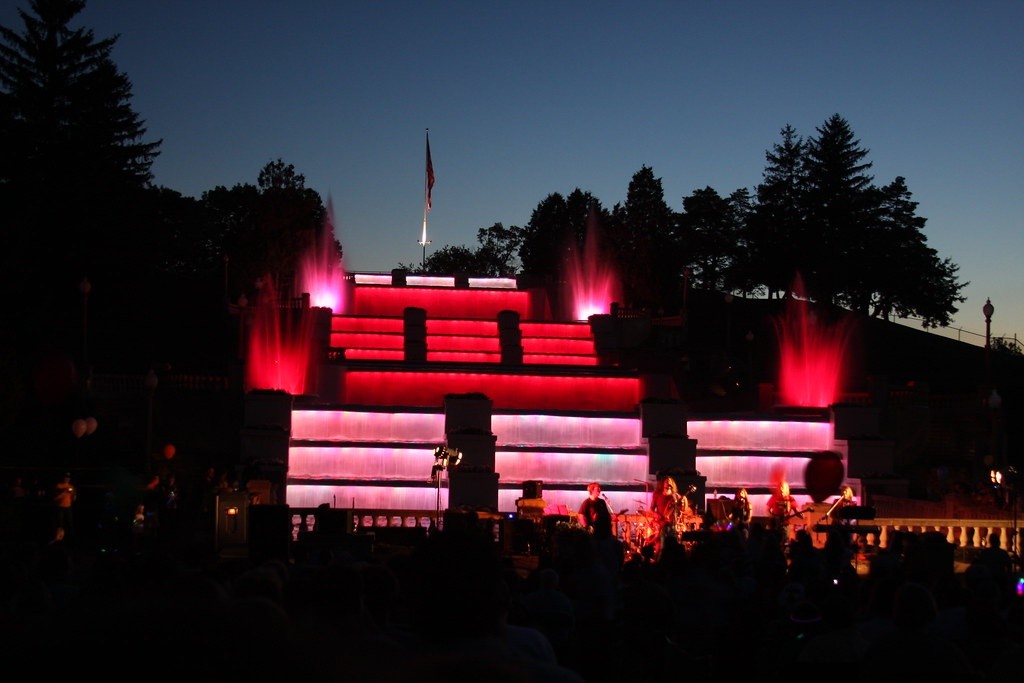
[85,417,98,436]
[162,443,177,461]
[72,419,88,439]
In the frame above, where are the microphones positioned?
[842,493,845,498]
[602,494,607,501]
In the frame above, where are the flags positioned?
[425,130,436,209]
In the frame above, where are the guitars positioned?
[766,510,816,530]
[664,483,697,521]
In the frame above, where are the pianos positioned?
[811,506,882,557]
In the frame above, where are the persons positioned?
[729,488,753,542]
[0,506,1022,683]
[830,486,860,545]
[577,482,612,539]
[649,473,689,562]
[930,450,1024,518]
[765,479,806,555]
[6,462,272,506]
